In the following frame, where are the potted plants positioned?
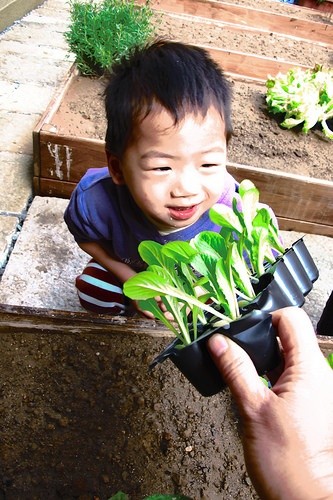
[121,179,321,398]
